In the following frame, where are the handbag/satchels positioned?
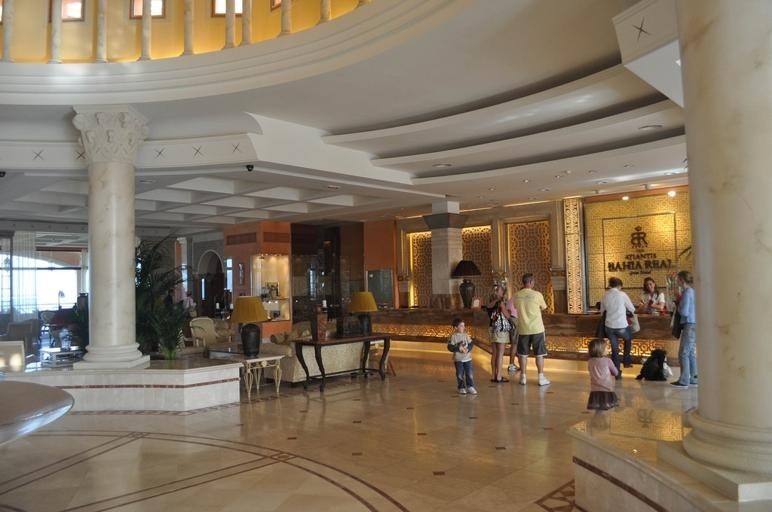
[595,310,607,338]
[626,312,640,334]
[494,311,512,332]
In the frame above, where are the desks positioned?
[291,334,390,392]
[229,351,285,396]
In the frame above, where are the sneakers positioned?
[539,377,550,385]
[671,381,689,387]
[491,364,526,385]
[457,387,478,394]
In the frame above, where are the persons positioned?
[512,272,551,386]
[506,297,520,372]
[637,277,666,314]
[182,290,197,318]
[587,338,621,430]
[163,288,175,307]
[670,271,698,388]
[486,285,512,383]
[448,318,478,395]
[600,277,636,369]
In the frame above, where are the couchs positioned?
[258,330,370,383]
[1,309,88,371]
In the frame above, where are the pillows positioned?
[268,330,337,344]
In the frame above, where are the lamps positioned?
[449,259,483,309]
[348,291,377,335]
[231,296,270,356]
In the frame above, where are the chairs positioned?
[187,316,238,353]
[174,329,207,358]
